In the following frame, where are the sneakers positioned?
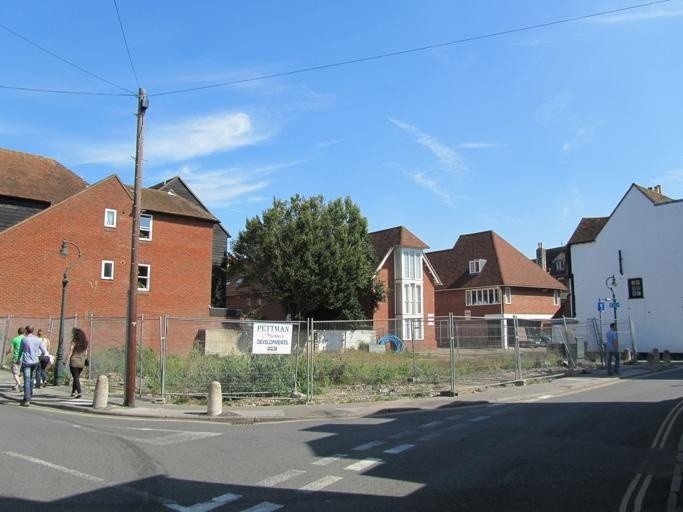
[607,368,621,376]
[12,380,82,407]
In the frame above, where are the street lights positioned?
[605,274,619,321]
[54,239,81,386]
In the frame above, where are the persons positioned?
[5,326,26,392]
[606,323,620,376]
[61,327,89,398]
[35,328,51,388]
[16,324,46,406]
[22,328,34,388]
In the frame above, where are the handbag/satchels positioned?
[45,354,55,370]
[85,359,90,366]
